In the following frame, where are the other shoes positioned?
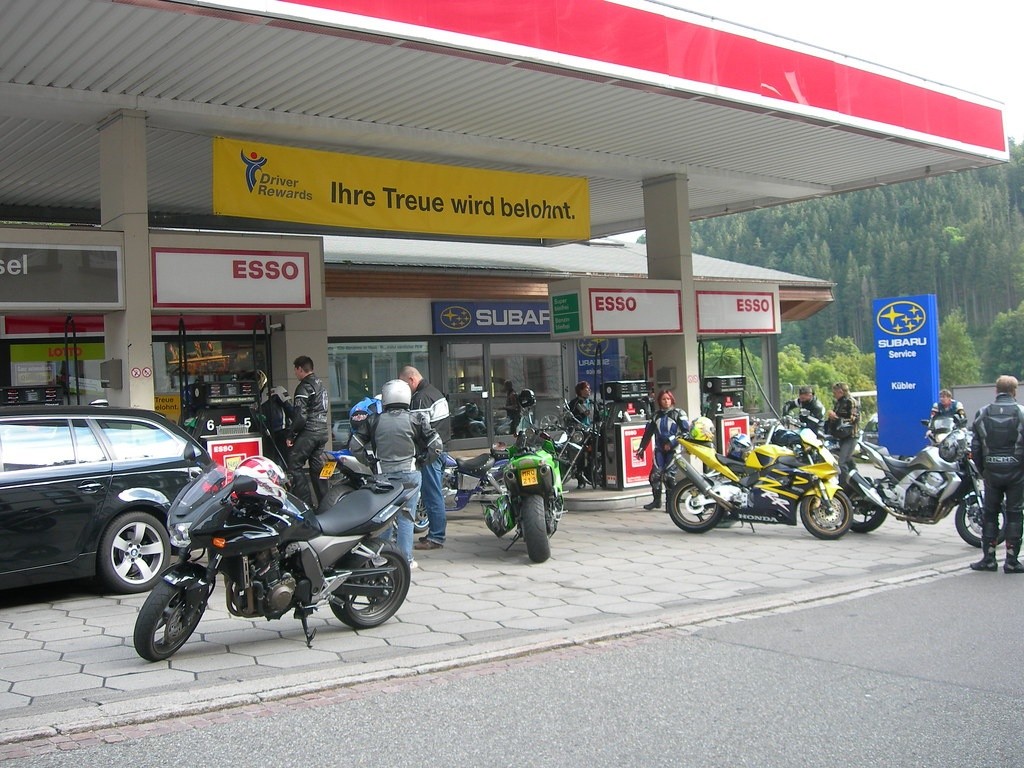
[409,560,419,570]
[577,482,585,490]
[415,539,442,550]
[419,536,427,542]
[666,503,669,513]
[643,499,662,510]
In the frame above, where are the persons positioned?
[370,378,443,569]
[568,381,591,488]
[399,365,452,549]
[970,375,1024,573]
[926,389,966,436]
[249,370,294,464]
[828,382,863,489]
[636,390,690,512]
[498,380,521,435]
[782,384,825,440]
[286,355,328,511]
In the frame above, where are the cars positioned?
[0,397,221,595]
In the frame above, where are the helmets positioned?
[379,380,411,412]
[466,403,478,418]
[689,416,716,442]
[520,388,536,407]
[351,396,384,444]
[938,428,967,463]
[233,455,290,511]
[726,433,751,460]
[485,494,515,538]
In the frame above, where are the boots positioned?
[969,539,998,572]
[1003,540,1024,574]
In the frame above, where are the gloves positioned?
[513,445,537,457]
[362,474,394,493]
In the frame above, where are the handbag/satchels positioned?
[831,417,853,439]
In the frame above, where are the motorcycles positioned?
[921,413,968,445]
[659,410,853,541]
[133,440,421,663]
[842,408,1011,549]
[449,399,486,437]
[461,380,606,563]
[317,395,458,535]
